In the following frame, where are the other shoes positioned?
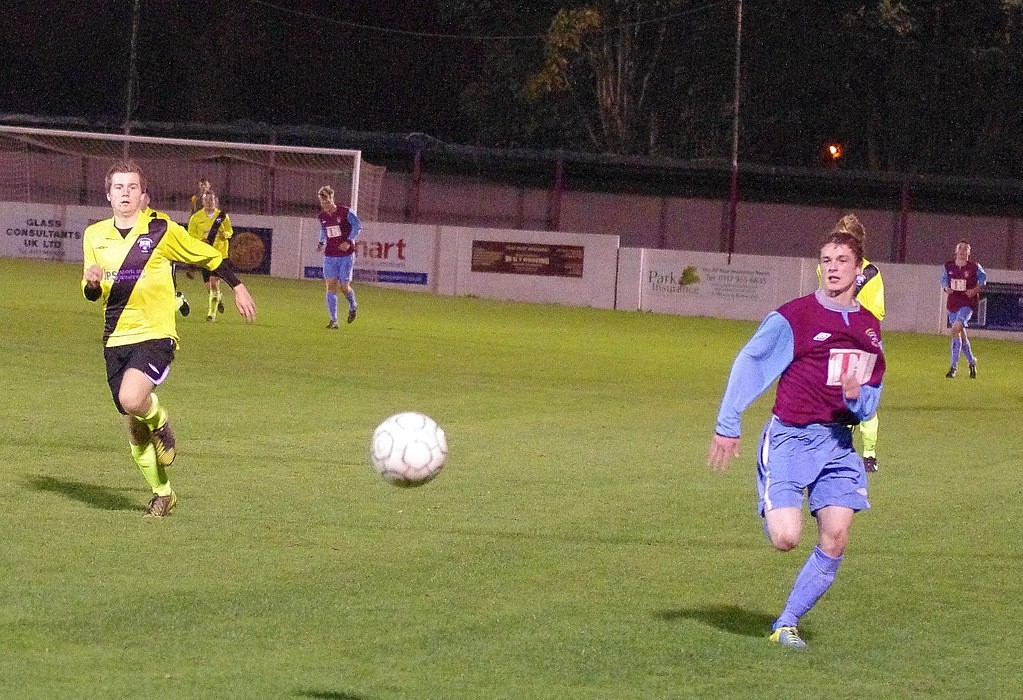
[186,270,196,280]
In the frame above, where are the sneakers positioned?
[969,366,977,379]
[153,420,176,466]
[142,488,178,518]
[946,369,957,379]
[326,321,338,330]
[177,291,191,318]
[863,457,879,472]
[215,301,224,313]
[207,315,216,322]
[348,309,357,324]
[769,626,805,649]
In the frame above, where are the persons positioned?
[186,177,220,280]
[81,161,259,518]
[315,184,361,330]
[187,191,234,323]
[815,214,886,473]
[940,239,987,378]
[141,187,192,318]
[708,234,887,646]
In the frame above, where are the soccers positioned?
[371,412,446,487]
